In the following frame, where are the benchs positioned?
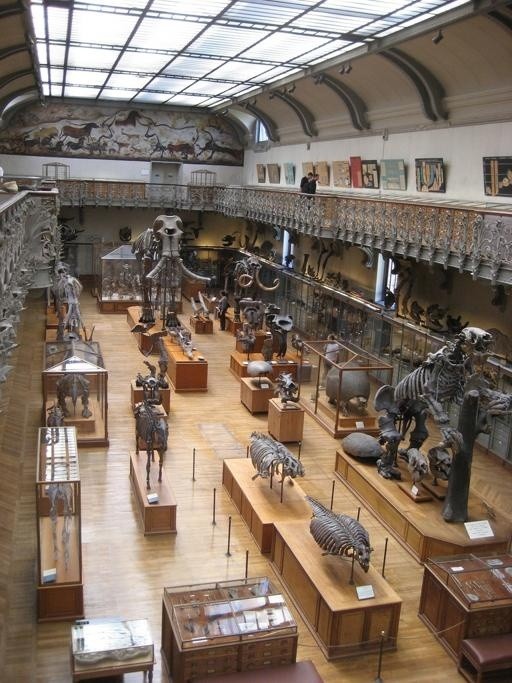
[184,660,325,683]
[456,634,512,683]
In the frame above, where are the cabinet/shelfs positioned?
[163,576,299,683]
[69,618,156,683]
[416,552,512,658]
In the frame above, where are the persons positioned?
[217,290,228,330]
[319,334,340,388]
[301,179,313,198]
[300,172,313,188]
[306,174,320,211]
